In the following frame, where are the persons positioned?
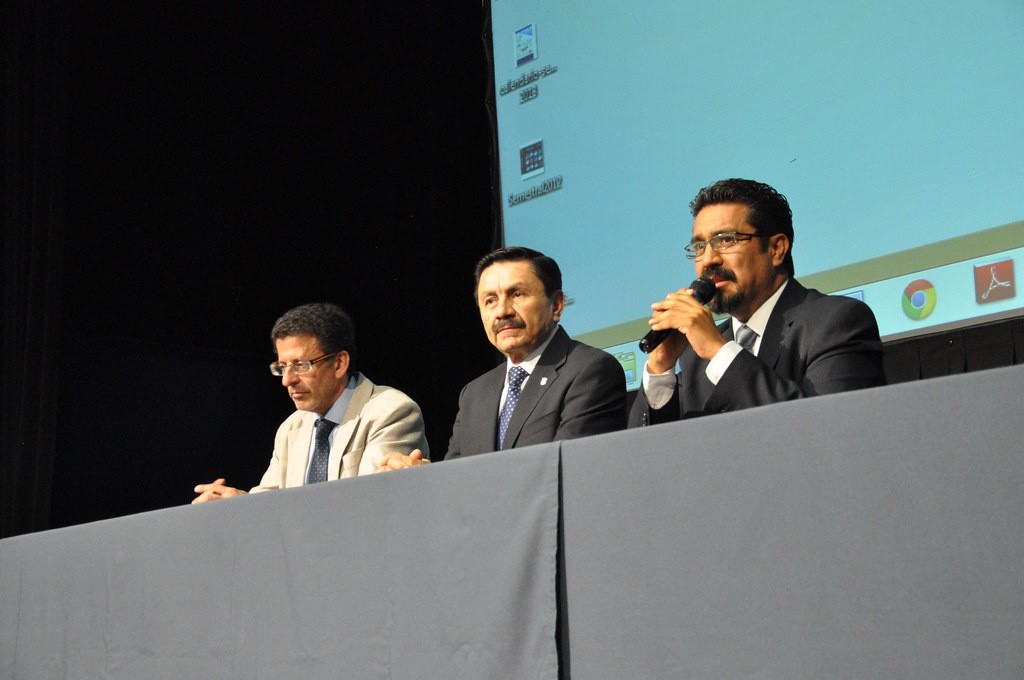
[192,304,429,504]
[377,246,628,473]
[628,178,888,428]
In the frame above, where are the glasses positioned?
[270,351,340,376]
[684,231,775,260]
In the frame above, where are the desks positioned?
[0,364,1024,679]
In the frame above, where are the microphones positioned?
[639,276,716,353]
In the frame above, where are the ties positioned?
[305,418,338,486]
[736,324,758,354]
[496,366,528,451]
